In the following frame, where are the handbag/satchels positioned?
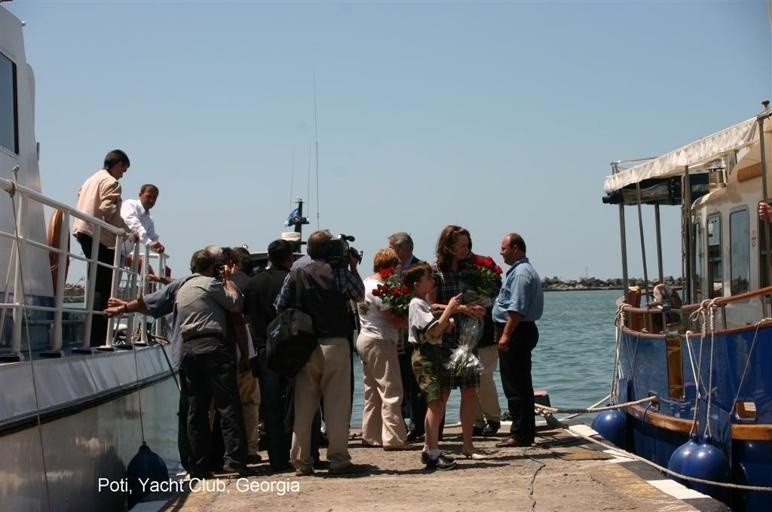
[178,332,228,376]
[265,267,317,377]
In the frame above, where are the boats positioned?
[591,100,772,512]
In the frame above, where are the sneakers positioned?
[422,451,429,464]
[328,463,361,476]
[295,467,315,476]
[425,453,456,471]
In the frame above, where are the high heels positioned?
[462,446,490,460]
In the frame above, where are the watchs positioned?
[115,307,121,315]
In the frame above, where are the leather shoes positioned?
[246,454,262,464]
[276,462,293,471]
[496,438,519,447]
[481,420,501,437]
[362,439,368,446]
[408,431,422,441]
[439,433,444,442]
[224,462,252,475]
[472,426,482,435]
[315,461,331,469]
[190,472,213,480]
[383,444,414,451]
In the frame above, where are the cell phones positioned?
[460,287,466,295]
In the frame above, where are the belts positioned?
[320,332,345,338]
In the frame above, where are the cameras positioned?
[328,233,363,265]
[217,265,226,284]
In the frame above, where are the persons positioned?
[403,265,464,472]
[104,244,222,320]
[204,245,261,465]
[230,248,250,309]
[274,231,365,476]
[386,232,433,443]
[491,233,544,447]
[243,238,321,471]
[471,251,502,438]
[119,185,164,295]
[170,248,259,480]
[72,149,138,352]
[107,298,174,319]
[435,225,488,462]
[356,248,416,451]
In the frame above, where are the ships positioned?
[2,32,187,512]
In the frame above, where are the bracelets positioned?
[123,304,128,313]
